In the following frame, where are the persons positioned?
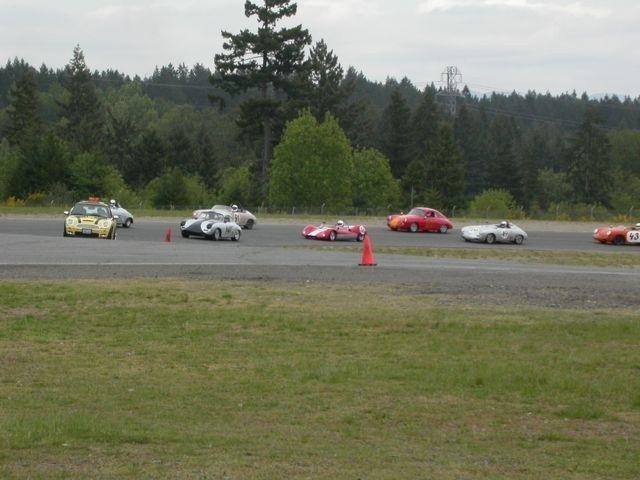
[337,219,344,228]
[109,198,117,209]
[634,222,640,230]
[231,204,239,212]
[499,220,508,229]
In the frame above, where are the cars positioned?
[593,225,639,246]
[212,203,212,204]
[192,204,258,229]
[180,209,242,241]
[462,221,528,244]
[83,198,133,228]
[387,207,453,234]
[63,197,116,240]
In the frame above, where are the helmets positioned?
[337,220,343,226]
[233,205,237,211]
[501,221,507,228]
[635,222,640,229]
[110,200,116,206]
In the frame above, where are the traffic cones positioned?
[359,236,379,267]
[165,227,174,243]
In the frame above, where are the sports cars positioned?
[302,220,370,241]
